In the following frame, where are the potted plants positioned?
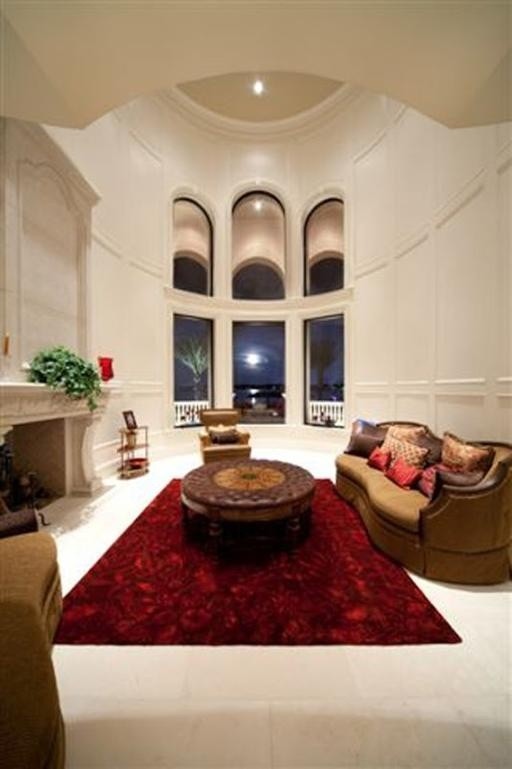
[24,345,103,414]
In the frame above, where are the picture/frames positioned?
[123,410,137,429]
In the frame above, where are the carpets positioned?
[44,476,464,647]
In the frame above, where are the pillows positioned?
[208,425,240,445]
[342,419,495,498]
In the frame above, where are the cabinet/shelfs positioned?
[118,426,149,479]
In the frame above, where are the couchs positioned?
[199,408,251,464]
[0,501,65,769]
[334,419,512,585]
